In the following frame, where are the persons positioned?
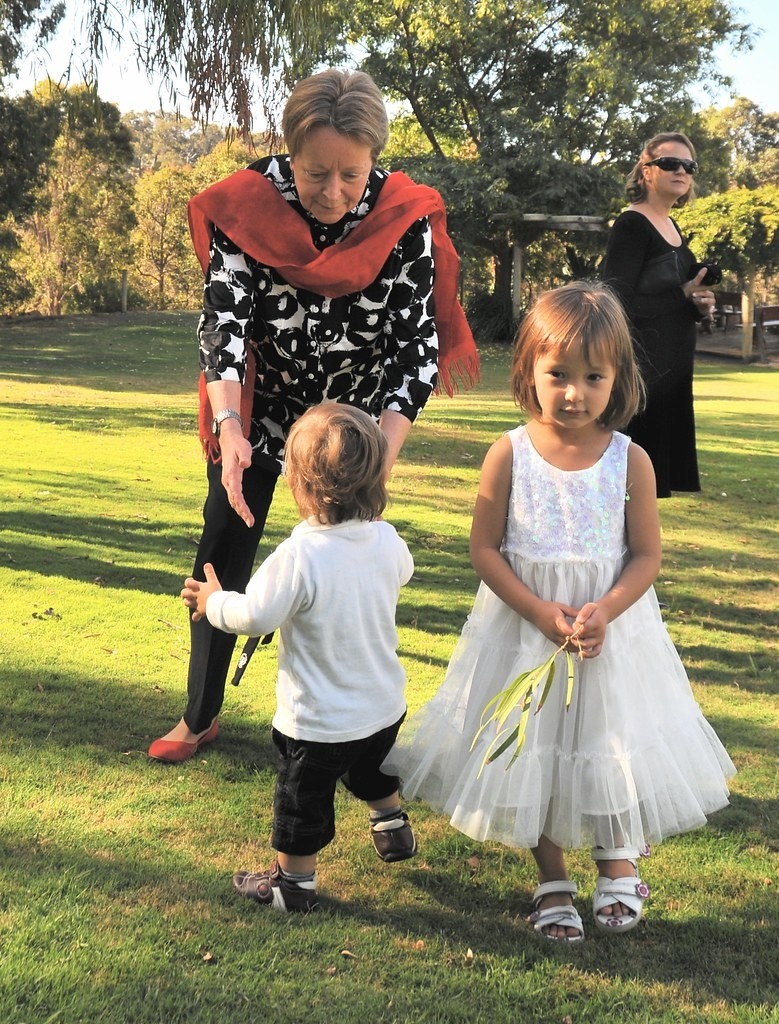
[150,67,483,762]
[382,277,738,942]
[180,402,416,916]
[599,130,717,501]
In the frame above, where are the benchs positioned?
[713,290,741,334]
[735,305,779,364]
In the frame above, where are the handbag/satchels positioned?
[634,251,681,295]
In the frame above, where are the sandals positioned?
[590,839,651,933]
[524,881,584,944]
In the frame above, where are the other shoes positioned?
[148,713,219,763]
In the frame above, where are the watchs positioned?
[211,409,244,438]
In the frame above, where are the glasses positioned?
[642,156,699,176]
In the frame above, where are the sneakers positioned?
[232,861,319,916]
[368,809,419,862]
